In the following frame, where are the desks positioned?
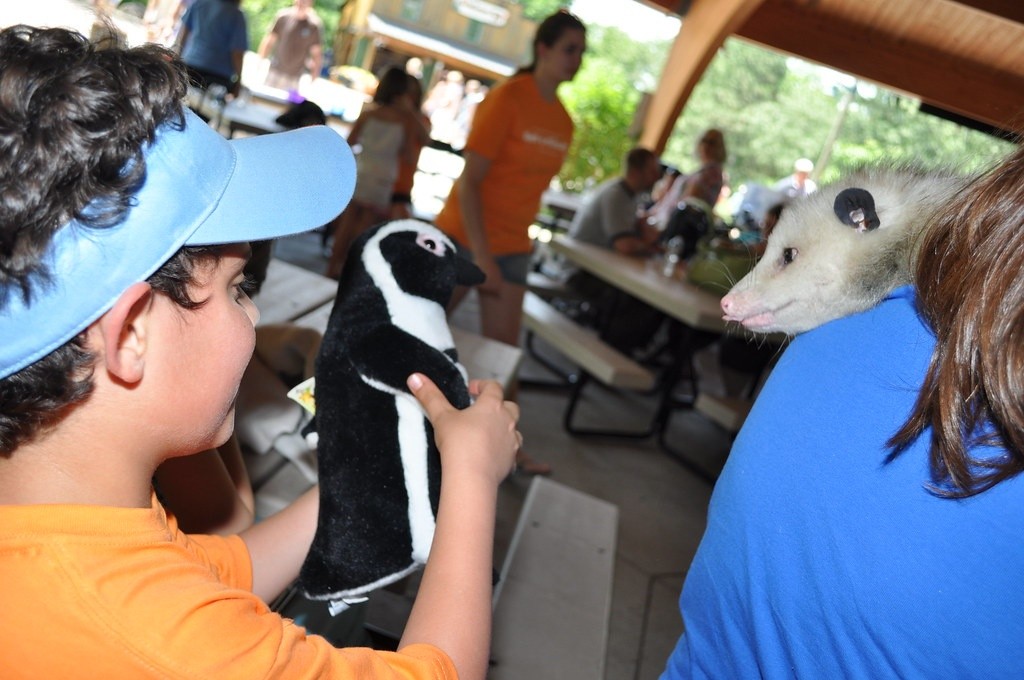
[547,232,794,461]
[245,260,524,614]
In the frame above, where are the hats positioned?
[0,101,358,384]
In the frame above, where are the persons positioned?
[1,23,523,680]
[672,202,789,402]
[166,0,249,121]
[430,9,585,474]
[568,129,726,376]
[405,57,485,136]
[326,64,432,277]
[660,149,1023,680]
[259,0,324,93]
[774,159,818,200]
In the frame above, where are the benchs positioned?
[522,287,655,437]
[478,473,618,680]
[694,388,753,434]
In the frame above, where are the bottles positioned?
[656,230,684,276]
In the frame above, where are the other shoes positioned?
[516,448,553,478]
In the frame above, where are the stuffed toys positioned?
[296,217,487,602]
